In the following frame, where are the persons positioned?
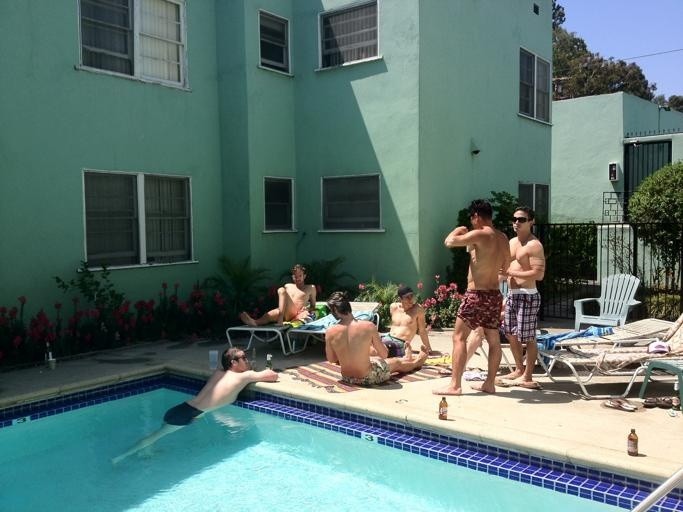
[238,262,316,327]
[104,346,279,465]
[429,199,513,396]
[368,285,443,357]
[323,290,428,387]
[496,204,546,387]
[437,295,509,375]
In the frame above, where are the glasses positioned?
[235,355,247,361]
[510,216,530,223]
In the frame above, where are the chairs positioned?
[479,272,682,408]
[224,298,381,355]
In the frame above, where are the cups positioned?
[209,350,218,369]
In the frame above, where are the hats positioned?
[397,287,412,298]
[648,342,671,353]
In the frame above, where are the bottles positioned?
[671,381,681,411]
[627,428,638,456]
[249,348,257,371]
[265,354,272,370]
[439,396,448,420]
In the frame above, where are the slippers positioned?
[657,396,673,408]
[605,398,637,412]
[643,397,657,407]
